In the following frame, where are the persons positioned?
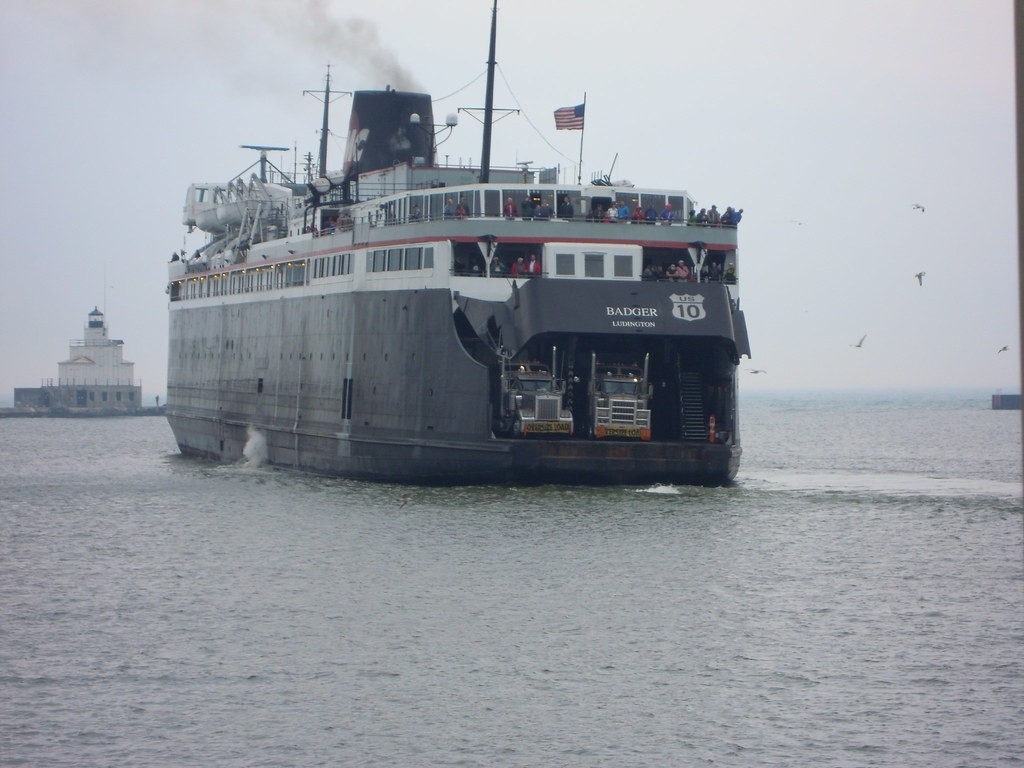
[504,194,575,222]
[304,215,353,238]
[443,197,470,219]
[640,260,736,284]
[409,205,420,223]
[687,205,743,227]
[587,202,673,225]
[455,252,541,277]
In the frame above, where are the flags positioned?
[554,102,585,131]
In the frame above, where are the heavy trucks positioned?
[576,352,654,441]
[461,339,573,438]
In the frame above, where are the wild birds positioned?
[912,202,925,213]
[850,335,868,346]
[746,368,765,375]
[999,345,1010,354]
[916,272,926,285]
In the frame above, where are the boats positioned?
[193,201,227,235]
[167,1,754,488]
[216,201,263,227]
